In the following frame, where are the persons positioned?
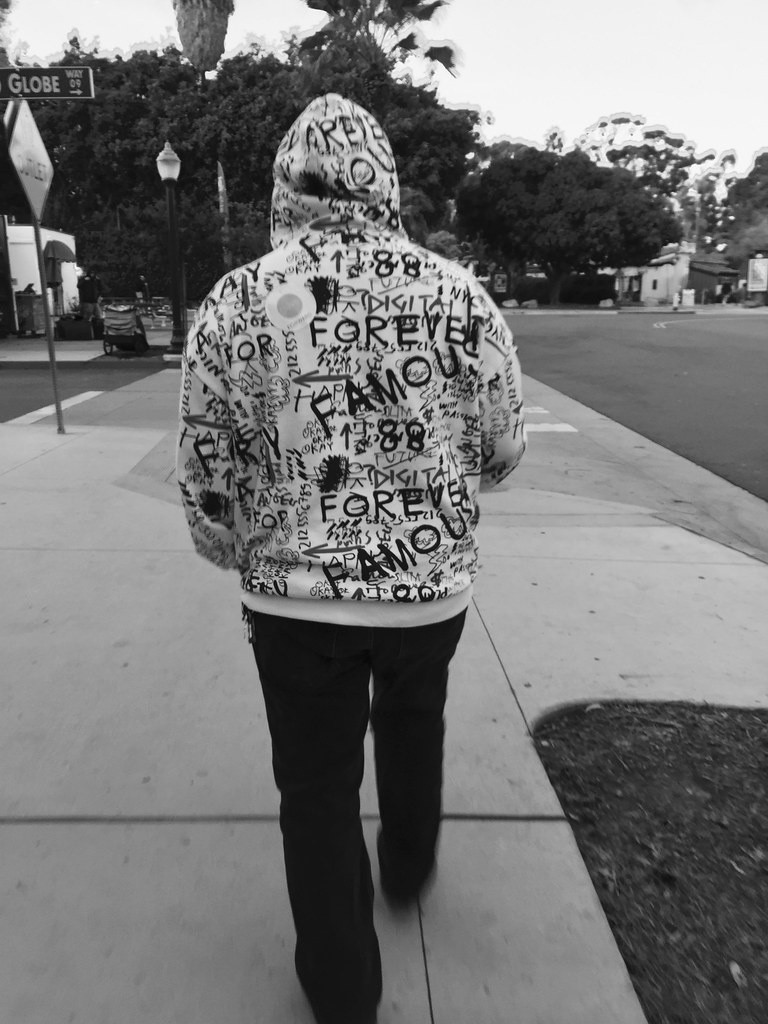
[177,94,526,1024]
